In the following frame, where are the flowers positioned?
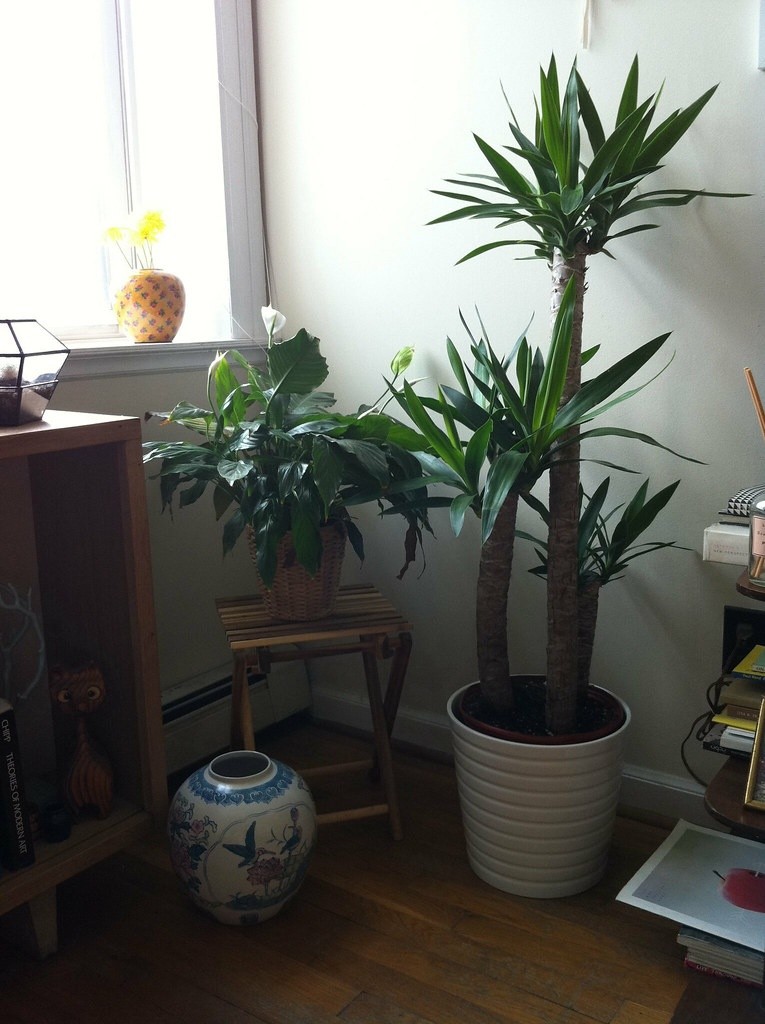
[108,214,165,269]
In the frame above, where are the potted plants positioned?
[141,303,442,623]
[382,50,756,899]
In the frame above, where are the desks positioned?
[216,584,416,844]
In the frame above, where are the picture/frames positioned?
[744,699,764,810]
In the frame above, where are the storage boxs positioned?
[703,487,765,566]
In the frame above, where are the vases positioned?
[112,272,186,343]
[168,750,317,925]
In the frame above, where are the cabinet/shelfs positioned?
[671,567,764,1024]
[0,408,169,959]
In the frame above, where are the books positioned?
[0,698,36,873]
[676,926,765,992]
[702,644,765,760]
[702,484,765,566]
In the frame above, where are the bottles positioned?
[748,491,765,588]
